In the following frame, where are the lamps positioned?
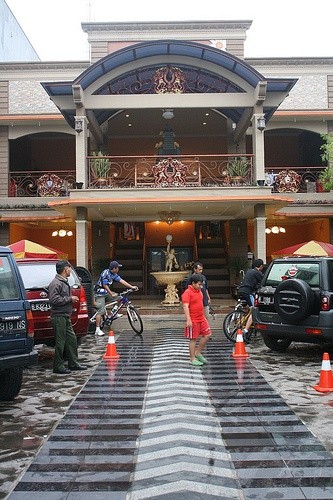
[74,119,83,135]
[162,112,174,120]
[257,116,266,134]
[266,217,285,234]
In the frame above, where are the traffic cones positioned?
[310,352,333,392]
[104,359,118,386]
[231,329,249,357]
[103,330,120,359]
[232,356,248,384]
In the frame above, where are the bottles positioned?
[111,304,118,314]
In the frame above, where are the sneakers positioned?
[242,329,251,344]
[95,329,105,336]
[110,311,123,319]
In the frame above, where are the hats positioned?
[56,260,71,268]
[110,261,123,268]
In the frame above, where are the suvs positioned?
[16,258,89,348]
[251,257,333,351]
[0,247,40,400]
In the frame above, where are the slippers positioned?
[195,355,208,364]
[189,360,203,366]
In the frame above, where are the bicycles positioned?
[88,287,143,334]
[223,299,257,344]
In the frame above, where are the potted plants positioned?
[228,156,248,184]
[92,151,110,186]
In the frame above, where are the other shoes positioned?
[68,365,88,370]
[53,369,71,374]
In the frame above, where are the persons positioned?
[181,262,212,366]
[48,260,87,374]
[165,243,178,272]
[238,258,265,344]
[93,261,138,335]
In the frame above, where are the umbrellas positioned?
[272,240,333,257]
[6,240,69,259]
